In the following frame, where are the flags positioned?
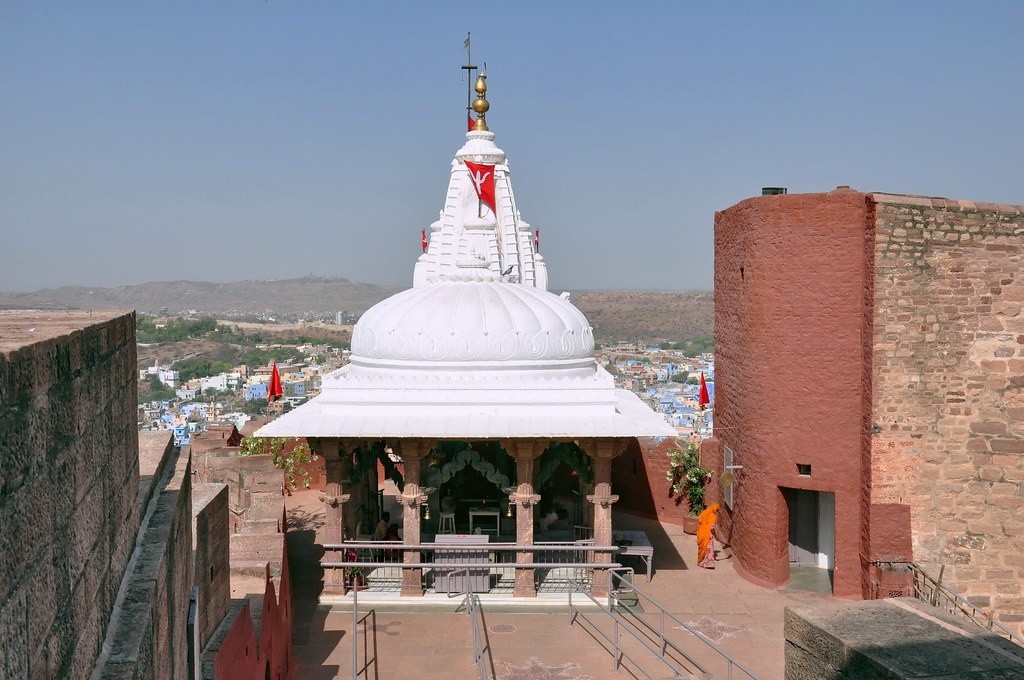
[699,374,710,406]
[464,160,496,214]
[535,229,539,245]
[267,363,283,402]
[422,231,427,249]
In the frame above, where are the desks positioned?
[611,530,654,581]
[469,506,500,536]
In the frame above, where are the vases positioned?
[682,513,700,535]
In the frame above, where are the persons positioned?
[697,506,720,568]
[374,512,403,559]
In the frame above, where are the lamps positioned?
[421,503,432,520]
[506,502,516,518]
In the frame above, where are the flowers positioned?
[663,439,714,519]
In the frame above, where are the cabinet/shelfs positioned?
[439,512,456,535]
